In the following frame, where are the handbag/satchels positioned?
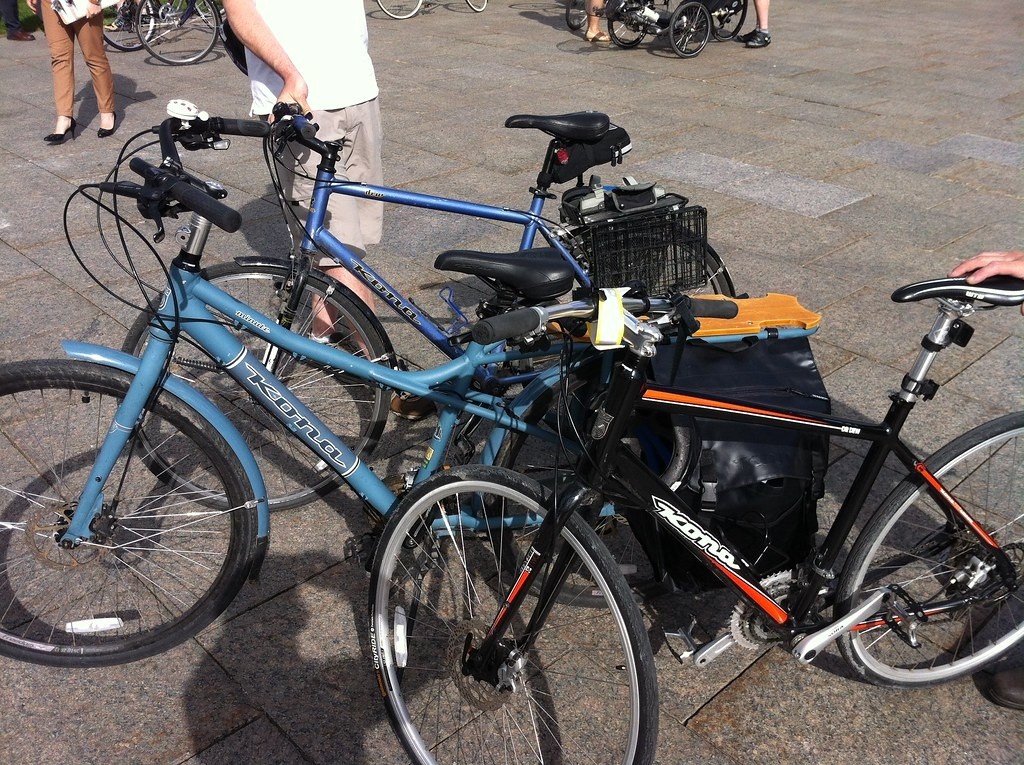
[50,1,122,26]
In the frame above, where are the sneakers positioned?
[293,333,402,390]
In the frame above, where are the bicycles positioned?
[566,0,640,32]
[102,0,228,66]
[0,102,820,669]
[376,0,487,20]
[363,269,1024,765]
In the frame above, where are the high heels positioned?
[97,112,117,139]
[43,117,78,143]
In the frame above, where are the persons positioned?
[116,0,157,24]
[585,0,611,41]
[26,0,117,143]
[0,0,37,40]
[735,0,772,48]
[223,1,440,421]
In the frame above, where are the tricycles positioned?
[595,0,748,58]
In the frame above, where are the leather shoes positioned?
[734,27,771,48]
[586,30,612,42]
